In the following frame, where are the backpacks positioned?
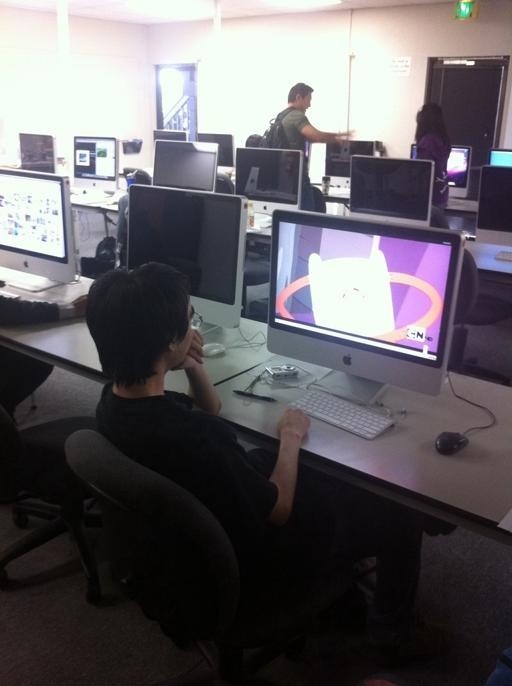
[256,106,296,150]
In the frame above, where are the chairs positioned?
[65,427,355,686]
[452,250,481,354]
[0,407,107,606]
[114,168,151,269]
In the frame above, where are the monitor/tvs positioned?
[349,155,435,227]
[0,168,76,284]
[266,209,465,396]
[196,133,233,167]
[235,146,304,216]
[152,138,219,192]
[126,184,248,328]
[324,140,376,188]
[73,135,119,191]
[487,148,512,165]
[410,144,472,198]
[152,129,188,144]
[476,165,512,246]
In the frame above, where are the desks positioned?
[0,274,273,395]
[214,355,512,562]
[55,172,511,274]
[214,165,479,212]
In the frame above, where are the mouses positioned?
[434,431,468,456]
[202,342,225,356]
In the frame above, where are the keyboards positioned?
[288,389,395,440]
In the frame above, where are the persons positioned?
[85,262,454,670]
[275,82,351,213]
[414,101,451,211]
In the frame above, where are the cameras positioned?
[266,365,298,378]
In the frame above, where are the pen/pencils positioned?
[232,389,278,402]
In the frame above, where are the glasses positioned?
[174,312,203,331]
